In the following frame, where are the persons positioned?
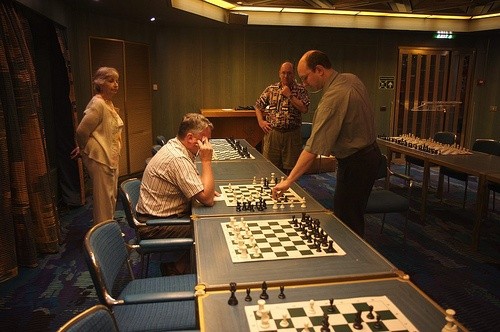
[272,50,380,239]
[255,62,311,176]
[70,67,124,226]
[136,113,215,242]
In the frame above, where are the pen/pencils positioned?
[196,138,206,157]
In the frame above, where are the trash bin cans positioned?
[302,121,312,146]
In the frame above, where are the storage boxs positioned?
[304,156,336,174]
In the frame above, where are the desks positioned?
[196,275,471,332]
[191,212,404,291]
[195,160,288,182]
[200,108,268,150]
[194,149,270,162]
[376,138,500,250]
[191,180,330,217]
[190,138,255,150]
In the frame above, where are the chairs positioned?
[404,132,457,193]
[83,219,200,332]
[439,139,500,209]
[57,304,120,332]
[119,178,195,278]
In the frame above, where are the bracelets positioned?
[288,94,293,99]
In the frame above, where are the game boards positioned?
[244,294,425,332]
[219,180,304,206]
[220,215,347,264]
[378,132,473,156]
[205,138,257,162]
